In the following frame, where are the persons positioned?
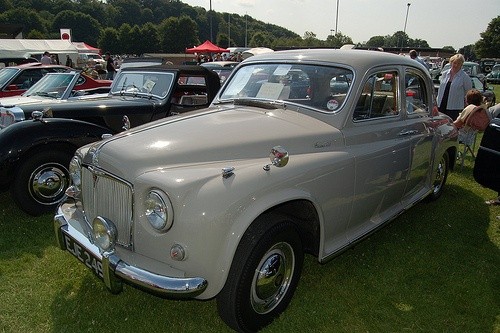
[160,58,168,66]
[196,52,243,66]
[436,53,472,122]
[116,53,130,61]
[432,54,454,74]
[454,88,483,147]
[105,52,115,80]
[409,49,429,72]
[41,51,76,69]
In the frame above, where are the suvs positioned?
[53,43,460,333]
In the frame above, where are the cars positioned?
[0,44,500,218]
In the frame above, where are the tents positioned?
[185,39,230,65]
[71,42,103,60]
[0,39,78,68]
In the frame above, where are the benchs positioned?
[180,85,207,95]
[332,95,395,114]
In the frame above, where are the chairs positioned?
[21,80,30,89]
[456,105,492,167]
